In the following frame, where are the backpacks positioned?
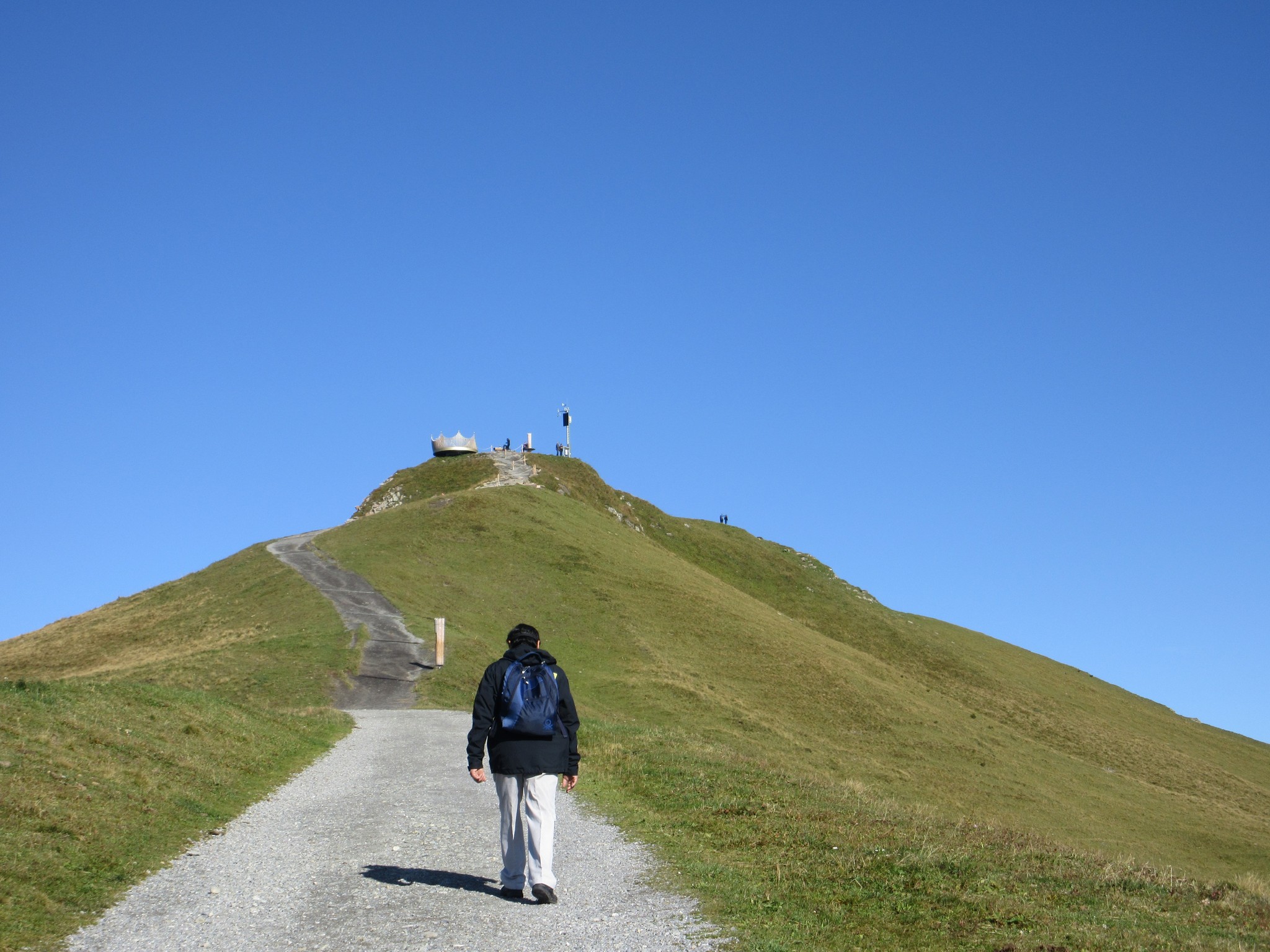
[495,657,559,736]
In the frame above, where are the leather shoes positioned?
[531,884,558,904]
[501,885,523,898]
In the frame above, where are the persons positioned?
[556,442,559,456]
[720,514,725,523]
[523,442,528,452]
[724,515,728,525]
[467,623,581,903]
[559,443,563,456]
[505,438,510,451]
